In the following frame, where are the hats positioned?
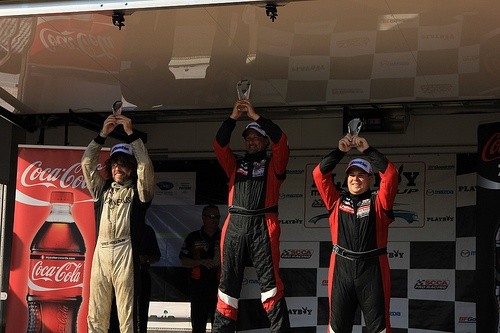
[110,143,135,157]
[242,122,270,139]
[345,159,373,174]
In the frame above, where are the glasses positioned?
[202,214,220,219]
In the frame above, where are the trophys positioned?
[347,118,362,147]
[236,80,252,111]
[112,100,123,126]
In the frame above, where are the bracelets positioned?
[146,255,149,265]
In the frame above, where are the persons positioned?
[108,190,161,333]
[81,115,154,333]
[214,99,290,333]
[313,134,399,333]
[179,205,222,333]
